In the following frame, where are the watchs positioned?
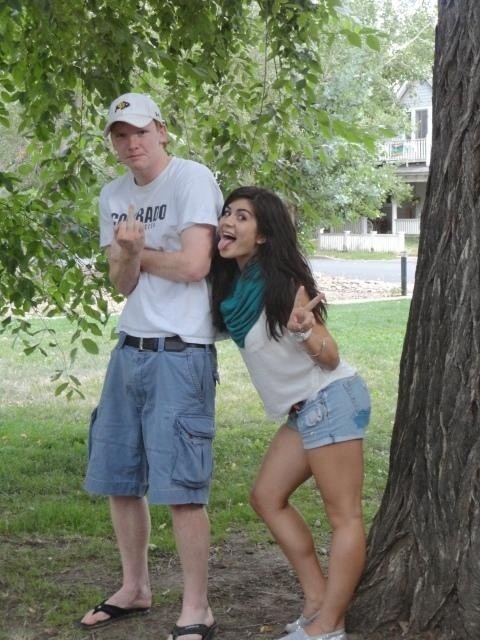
[286,327,313,343]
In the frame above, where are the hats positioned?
[102,92,163,138]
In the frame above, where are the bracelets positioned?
[308,335,327,358]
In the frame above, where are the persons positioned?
[207,186,373,639]
[76,90,230,638]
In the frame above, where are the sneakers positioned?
[279,610,347,639]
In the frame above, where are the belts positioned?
[124,335,214,352]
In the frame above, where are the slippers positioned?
[78,598,150,630]
[169,618,219,640]
